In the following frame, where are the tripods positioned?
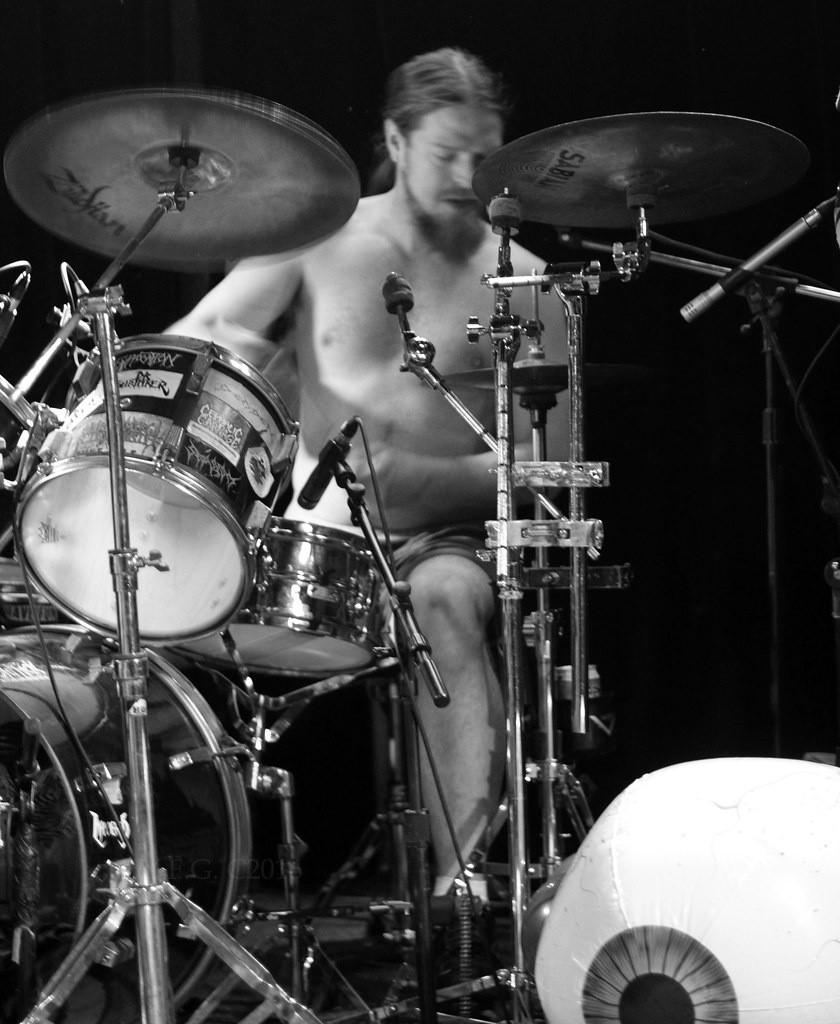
[17,162,596,1024]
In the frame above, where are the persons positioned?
[152,48,581,1019]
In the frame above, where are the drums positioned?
[0,558,74,640]
[0,619,255,1023]
[161,620,396,910]
[11,330,303,647]
[159,514,392,677]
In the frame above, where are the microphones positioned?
[297,412,362,511]
[0,269,30,347]
[678,197,840,323]
[73,278,121,348]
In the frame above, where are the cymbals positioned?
[2,86,364,277]
[472,108,812,231]
